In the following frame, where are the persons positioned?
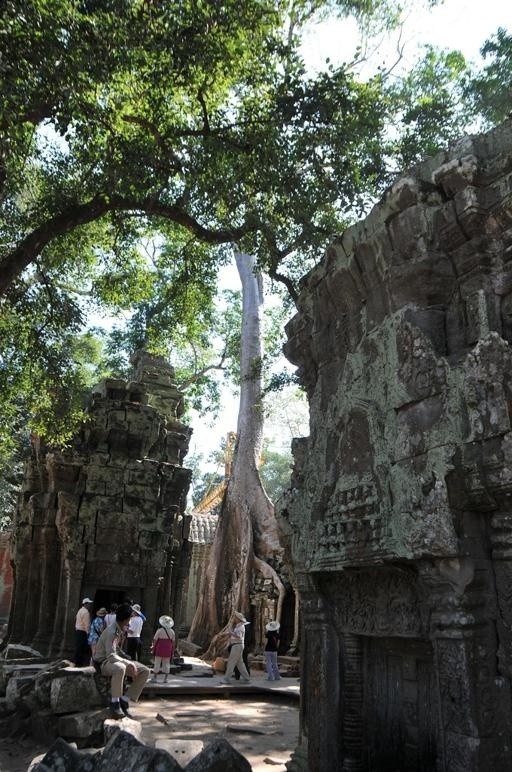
[228,617,251,681]
[91,602,149,719]
[217,611,251,685]
[73,596,147,667]
[264,620,284,679]
[151,615,177,683]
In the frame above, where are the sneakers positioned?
[279,676,282,679]
[238,678,252,684]
[218,678,232,685]
[263,676,275,681]
[148,678,158,683]
[162,679,169,683]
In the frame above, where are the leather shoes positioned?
[109,701,124,719]
[119,697,131,718]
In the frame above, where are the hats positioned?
[158,613,176,629]
[266,620,281,631]
[242,618,251,626]
[130,603,142,616]
[96,607,109,617]
[82,597,94,605]
[234,610,247,623]
